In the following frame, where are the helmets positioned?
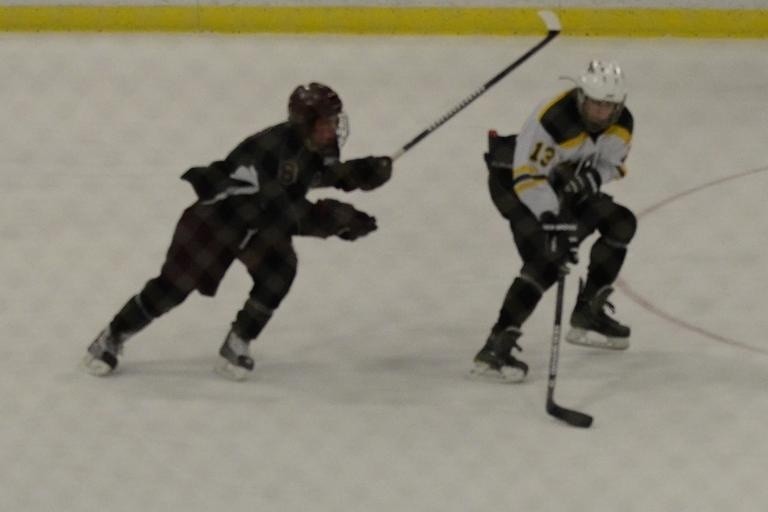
[287,80,349,156]
[556,54,629,104]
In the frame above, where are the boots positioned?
[219,317,258,369]
[472,313,532,372]
[86,317,126,367]
[570,279,630,337]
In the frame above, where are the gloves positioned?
[310,200,380,242]
[537,207,579,278]
[552,168,601,212]
[337,155,394,193]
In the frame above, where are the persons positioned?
[84,82,391,383]
[457,58,637,382]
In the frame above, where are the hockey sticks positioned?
[547,275,593,428]
[390,9,561,161]
